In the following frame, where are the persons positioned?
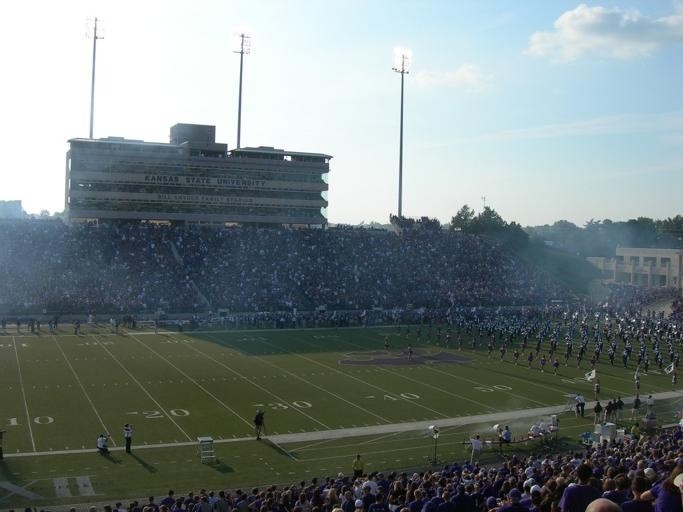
[0,218,683,334]
[253,409,265,440]
[383,336,391,353]
[351,454,364,483]
[97,434,111,456]
[123,423,135,453]
[1,471,401,512]
[402,335,683,511]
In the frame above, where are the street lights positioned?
[390,48,411,216]
[84,14,105,139]
[230,30,253,147]
